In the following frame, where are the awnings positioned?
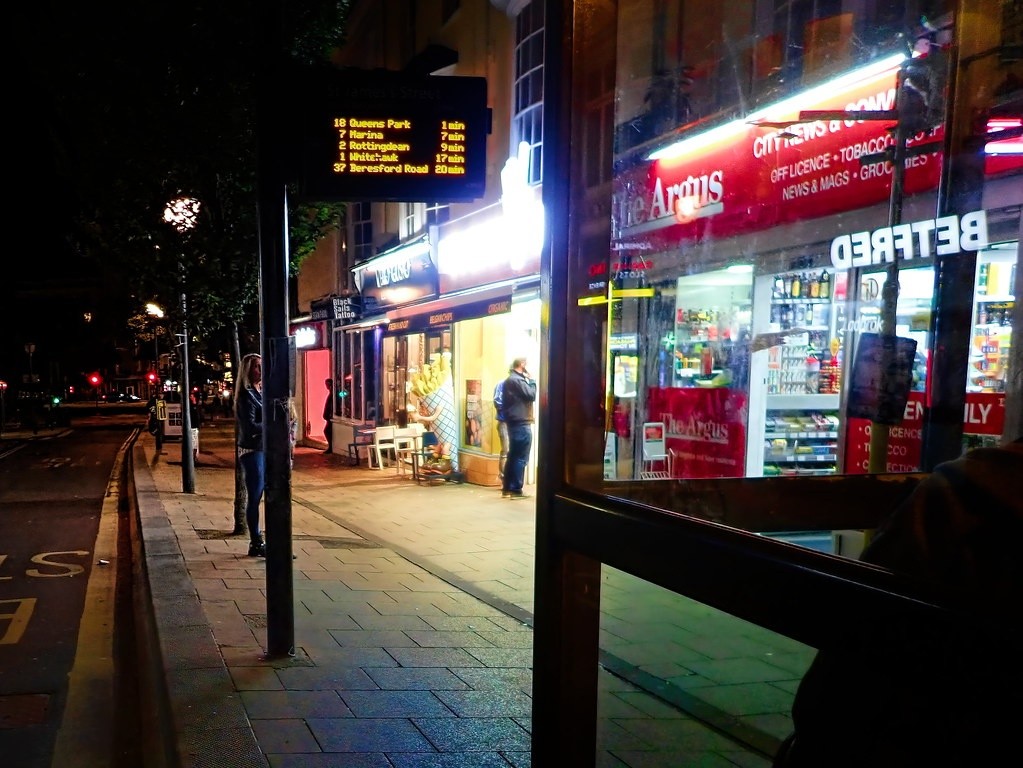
[309,292,360,323]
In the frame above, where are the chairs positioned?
[348,420,439,485]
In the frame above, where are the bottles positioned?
[773,268,830,299]
[772,304,814,327]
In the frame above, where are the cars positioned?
[103,391,141,403]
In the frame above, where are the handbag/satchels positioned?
[149,418,160,436]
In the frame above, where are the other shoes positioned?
[510,494,527,499]
[323,449,332,454]
[502,492,511,497]
[248,542,266,557]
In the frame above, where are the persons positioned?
[234,354,265,558]
[771,438,1023,768]
[145,391,168,455]
[190,385,208,428]
[213,394,234,418]
[290,399,298,470]
[322,379,333,455]
[493,358,537,500]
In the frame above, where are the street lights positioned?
[164,196,202,494]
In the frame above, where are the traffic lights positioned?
[149,373,154,380]
[52,397,60,406]
[92,376,98,383]
